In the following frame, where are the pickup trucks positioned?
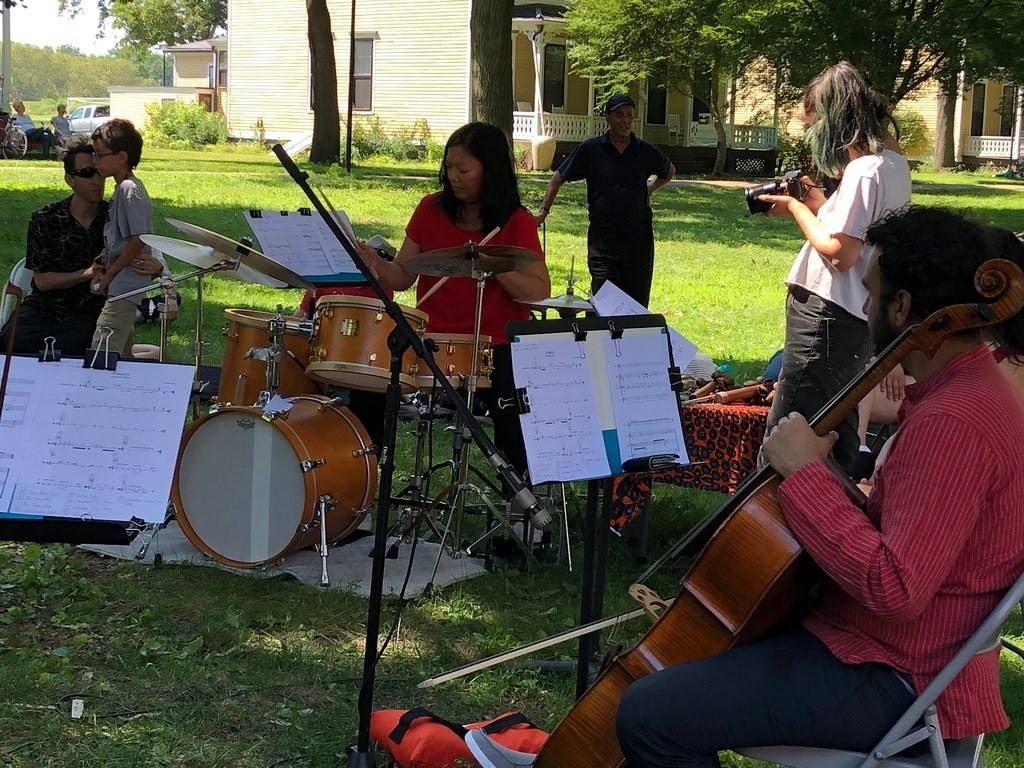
[68,104,110,137]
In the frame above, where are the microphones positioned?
[481,446,552,529]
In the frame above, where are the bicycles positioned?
[0,114,28,162]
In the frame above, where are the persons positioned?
[463,200,1024,768]
[5,100,73,157]
[534,94,676,310]
[0,118,181,358]
[755,60,911,475]
[349,122,554,551]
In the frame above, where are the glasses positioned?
[92,151,118,160]
[71,166,101,179]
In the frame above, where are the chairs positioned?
[517,101,533,112]
[667,114,685,145]
[0,255,34,330]
[698,112,712,124]
[732,572,1024,768]
[551,105,564,115]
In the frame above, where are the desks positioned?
[610,386,771,537]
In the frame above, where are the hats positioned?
[13,101,22,110]
[606,95,635,112]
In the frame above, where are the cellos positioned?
[531,258,1024,768]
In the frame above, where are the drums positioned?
[169,393,378,570]
[414,331,495,390]
[215,307,316,408]
[305,293,431,396]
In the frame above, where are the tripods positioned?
[369,255,548,575]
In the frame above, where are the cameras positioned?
[746,171,809,215]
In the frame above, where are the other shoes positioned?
[486,530,550,557]
[464,727,537,768]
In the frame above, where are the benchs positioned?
[0,120,86,158]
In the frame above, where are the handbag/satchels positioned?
[370,708,549,768]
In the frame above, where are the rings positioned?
[137,259,144,266]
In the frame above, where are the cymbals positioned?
[512,294,595,312]
[402,244,541,278]
[139,234,289,288]
[163,217,318,291]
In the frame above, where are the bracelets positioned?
[538,205,550,213]
[151,264,164,282]
